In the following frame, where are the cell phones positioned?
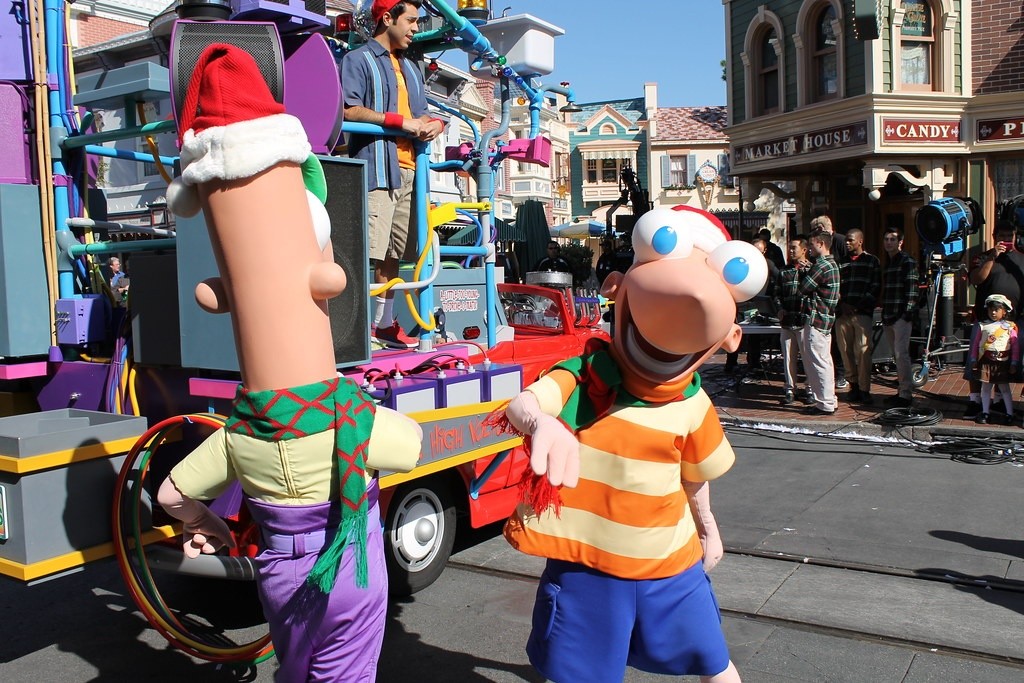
[1000,242,1014,251]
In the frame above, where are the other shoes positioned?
[783,395,796,404]
[962,401,983,420]
[726,360,740,372]
[979,412,990,425]
[803,405,831,415]
[806,395,817,404]
[859,391,874,405]
[1004,414,1013,426]
[883,397,912,409]
[747,362,763,374]
[849,388,861,401]
[882,393,900,404]
[989,399,1016,416]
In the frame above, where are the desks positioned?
[738,317,781,333]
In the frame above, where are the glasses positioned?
[995,234,1013,239]
[114,263,121,268]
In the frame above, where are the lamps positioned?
[812,180,820,192]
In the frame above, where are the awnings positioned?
[495,217,526,242]
[447,225,477,245]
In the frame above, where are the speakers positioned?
[168,0,344,155]
[0,0,53,357]
[130,153,372,372]
[445,143,474,163]
[508,137,552,167]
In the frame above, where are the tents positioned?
[548,218,618,257]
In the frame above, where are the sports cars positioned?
[374,283,610,593]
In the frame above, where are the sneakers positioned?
[371,314,419,348]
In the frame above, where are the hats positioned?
[984,294,1013,312]
[372,0,423,25]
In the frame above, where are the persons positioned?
[723,215,881,417]
[538,240,570,273]
[106,256,130,308]
[158,42,422,683]
[596,233,634,291]
[961,220,1024,426]
[879,229,921,406]
[478,205,769,682]
[340,0,444,347]
